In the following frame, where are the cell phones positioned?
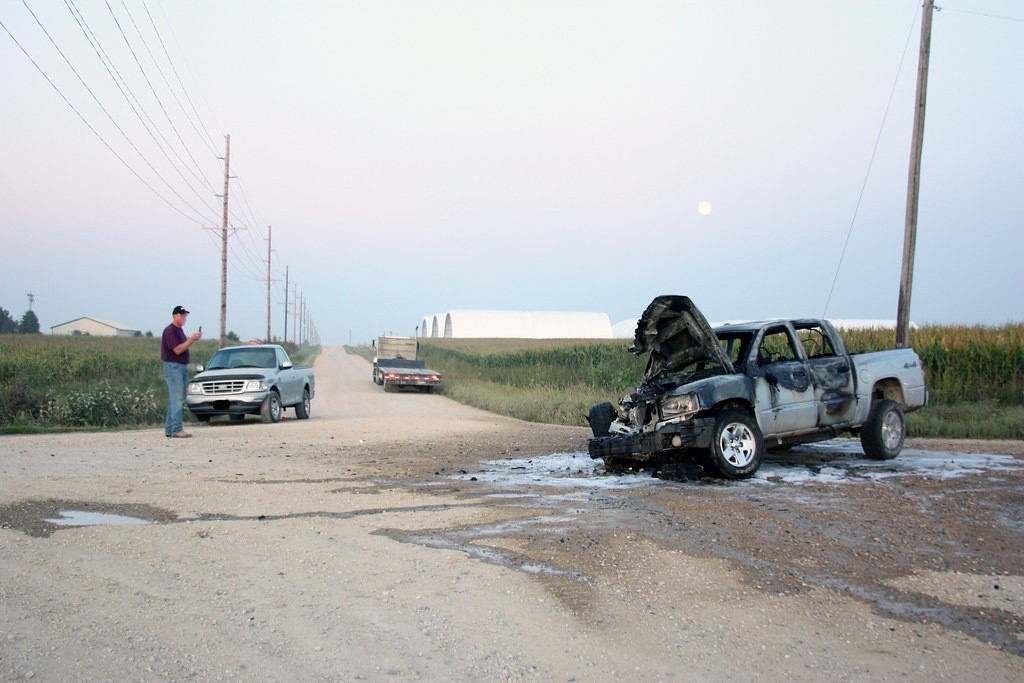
[199,326,202,333]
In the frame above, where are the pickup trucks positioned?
[586,294,929,483]
[185,344,317,424]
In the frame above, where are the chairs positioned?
[229,359,243,368]
[268,357,276,367]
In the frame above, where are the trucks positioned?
[372,335,443,395]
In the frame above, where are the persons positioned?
[161,306,202,439]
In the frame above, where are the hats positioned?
[173,306,189,314]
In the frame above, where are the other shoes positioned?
[166,433,171,437]
[172,431,191,438]
